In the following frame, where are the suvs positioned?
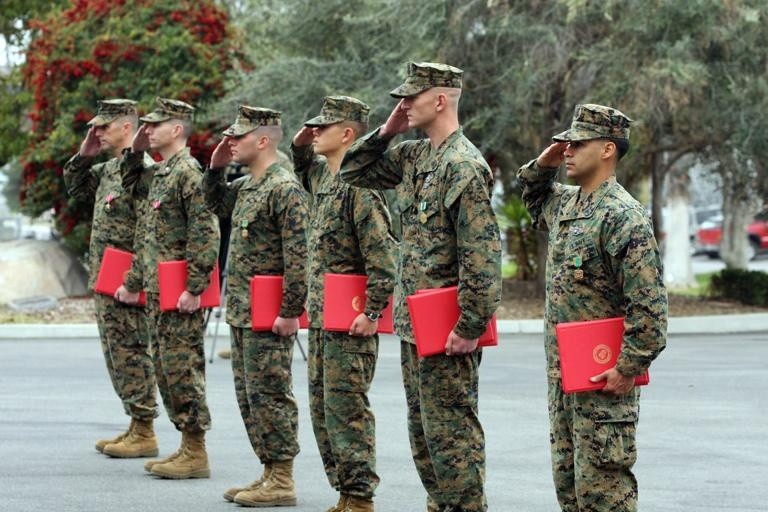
[688,205,724,239]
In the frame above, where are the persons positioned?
[118,93,222,482]
[61,98,161,459]
[516,102,667,511]
[339,59,505,512]
[200,102,310,510]
[288,95,398,512]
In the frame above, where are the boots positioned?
[222,458,297,506]
[142,432,211,480]
[324,492,375,512]
[94,416,160,458]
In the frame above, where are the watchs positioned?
[363,309,384,323]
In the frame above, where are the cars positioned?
[695,203,768,261]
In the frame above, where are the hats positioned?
[139,93,196,125]
[221,104,284,139]
[389,61,465,100]
[303,94,370,128]
[84,98,139,130]
[551,103,634,144]
